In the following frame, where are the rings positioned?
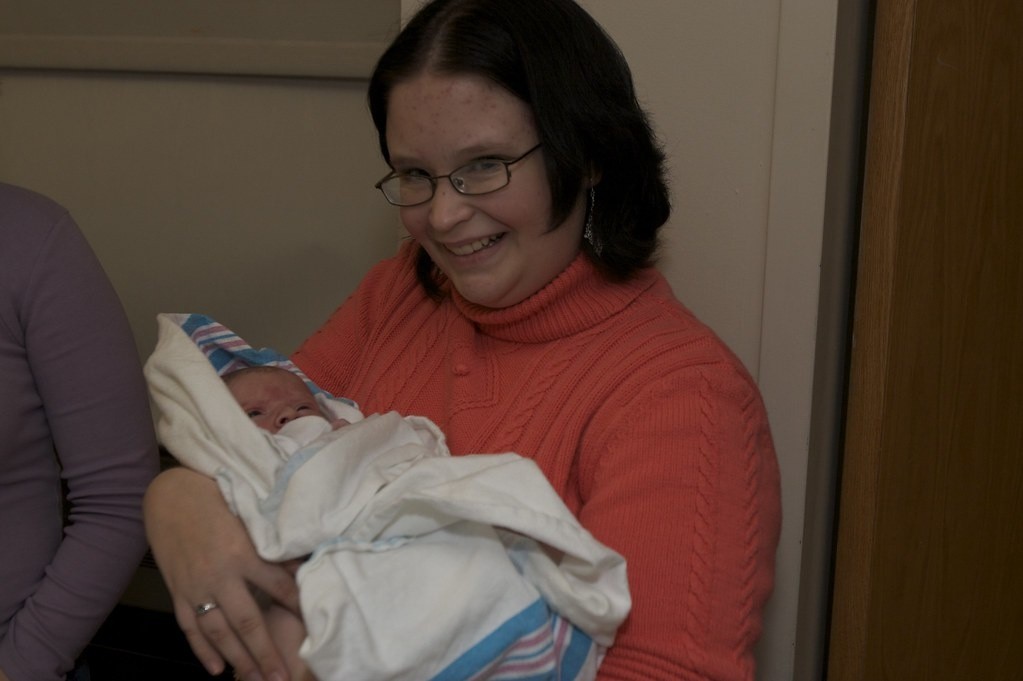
[195,602,218,617]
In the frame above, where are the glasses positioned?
[375,142,545,207]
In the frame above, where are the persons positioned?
[142,313,633,681]
[0,182,160,681]
[143,0,785,681]
